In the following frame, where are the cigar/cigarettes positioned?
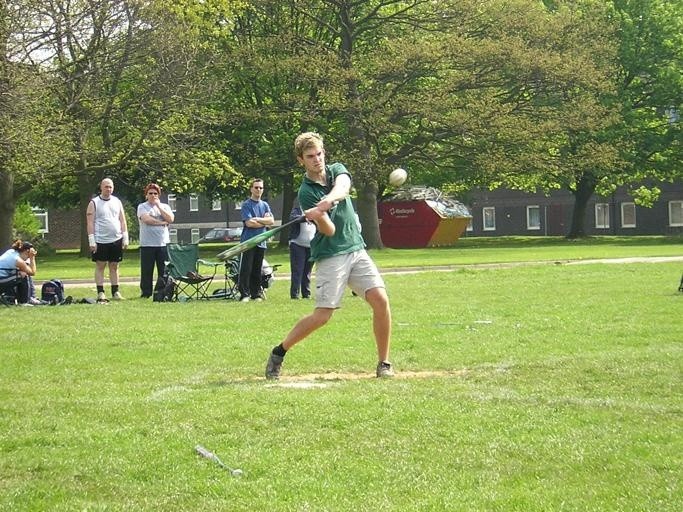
[93,250,96,253]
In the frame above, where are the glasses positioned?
[254,186,263,190]
[148,192,158,195]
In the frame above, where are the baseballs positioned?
[389,168,408,187]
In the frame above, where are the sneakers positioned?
[266,350,284,382]
[241,297,250,303]
[19,302,34,307]
[254,297,262,302]
[31,298,41,305]
[98,292,105,300]
[376,360,394,379]
[113,291,126,301]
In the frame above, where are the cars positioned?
[192,228,241,243]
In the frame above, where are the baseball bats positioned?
[216,201,339,263]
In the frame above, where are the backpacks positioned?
[41,278,65,305]
[153,272,176,303]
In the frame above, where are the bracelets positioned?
[87,235,97,247]
[122,231,130,246]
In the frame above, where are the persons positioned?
[85,177,129,303]
[238,178,274,301]
[264,132,395,380]
[0,240,37,307]
[287,197,316,300]
[136,184,175,298]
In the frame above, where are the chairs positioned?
[0,263,25,309]
[224,253,284,305]
[162,236,224,304]
